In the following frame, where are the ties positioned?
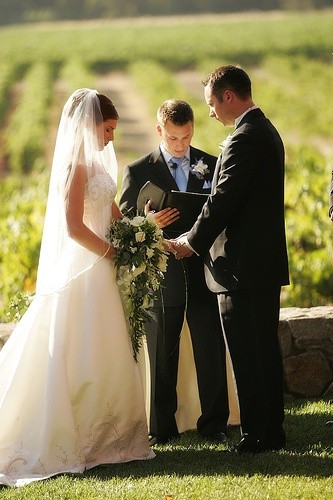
[169,156,187,192]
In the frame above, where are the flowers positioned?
[188,157,210,179]
[105,203,170,363]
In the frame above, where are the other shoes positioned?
[232,434,285,455]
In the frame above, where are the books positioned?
[137,181,211,228]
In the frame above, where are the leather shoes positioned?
[149,435,172,446]
[202,430,227,442]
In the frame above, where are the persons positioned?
[1,88,180,489]
[119,98,230,447]
[169,66,290,453]
